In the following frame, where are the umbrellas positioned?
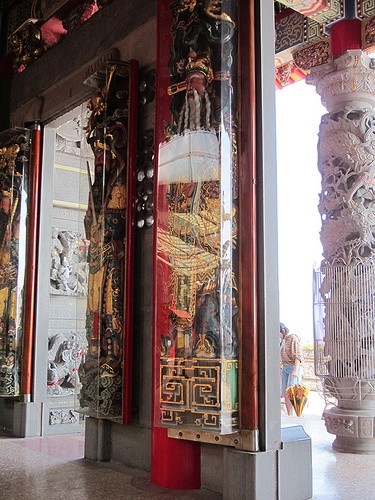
[284,376,310,417]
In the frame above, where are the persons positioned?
[279,323,303,416]
[162,40,238,360]
[79,133,127,378]
[0,143,28,367]
